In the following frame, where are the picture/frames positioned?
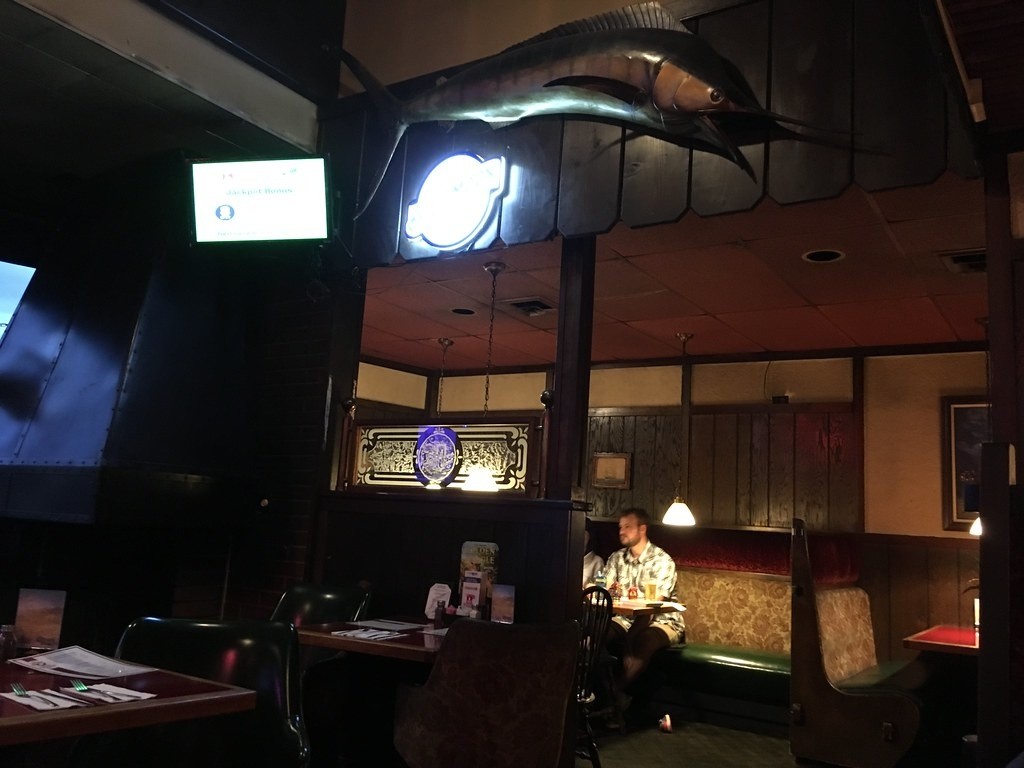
[591,451,632,490]
[939,393,991,532]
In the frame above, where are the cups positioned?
[644,579,657,601]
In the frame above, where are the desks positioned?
[901,624,980,658]
[297,615,453,663]
[584,597,685,737]
[0,650,259,748]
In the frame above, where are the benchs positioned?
[790,518,940,768]
[645,572,796,739]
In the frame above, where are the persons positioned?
[583,507,685,734]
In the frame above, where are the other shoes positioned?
[658,714,672,733]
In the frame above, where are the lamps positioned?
[661,333,700,528]
[461,261,506,494]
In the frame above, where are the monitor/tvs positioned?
[185,153,335,247]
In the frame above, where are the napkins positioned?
[60,682,159,705]
[0,688,94,712]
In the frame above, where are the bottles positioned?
[595,571,606,600]
[434,600,447,629]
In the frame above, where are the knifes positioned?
[89,687,141,698]
[35,689,91,706]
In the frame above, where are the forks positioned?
[367,629,397,638]
[11,683,59,708]
[71,679,120,701]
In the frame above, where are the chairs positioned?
[268,579,372,682]
[63,616,311,768]
[389,615,582,768]
[566,585,612,768]
